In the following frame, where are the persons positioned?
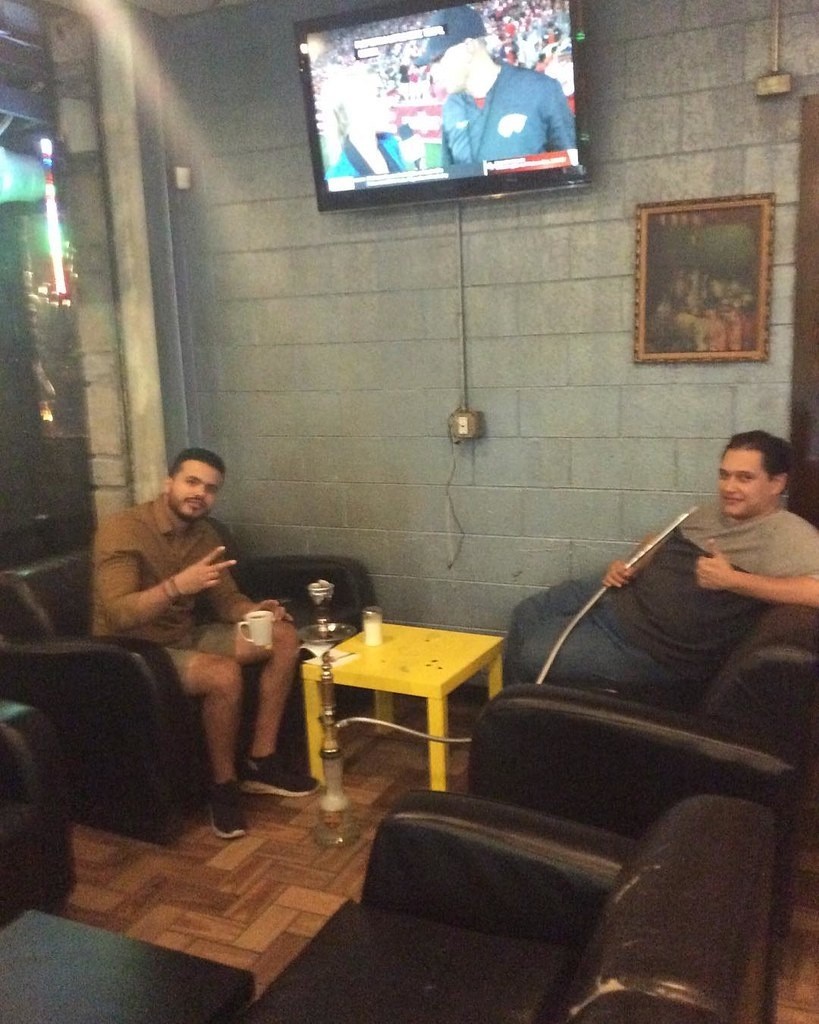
[502,430,819,710]
[321,0,575,180]
[93,447,321,839]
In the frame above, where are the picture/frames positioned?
[631,191,777,366]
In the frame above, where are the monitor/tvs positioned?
[293,0,592,214]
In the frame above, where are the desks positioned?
[302,623,507,793]
[0,906,259,1024]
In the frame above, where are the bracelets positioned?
[161,577,183,604]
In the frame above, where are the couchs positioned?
[233,783,780,1024]
[0,536,379,843]
[463,595,819,854]
[0,693,83,922]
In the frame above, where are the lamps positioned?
[756,0,793,97]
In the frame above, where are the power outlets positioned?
[458,417,469,436]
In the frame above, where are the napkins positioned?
[299,641,359,669]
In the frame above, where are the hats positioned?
[415,7,486,66]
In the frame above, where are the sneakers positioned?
[240,753,320,797]
[207,788,247,838]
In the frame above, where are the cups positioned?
[361,606,383,647]
[238,611,273,646]
